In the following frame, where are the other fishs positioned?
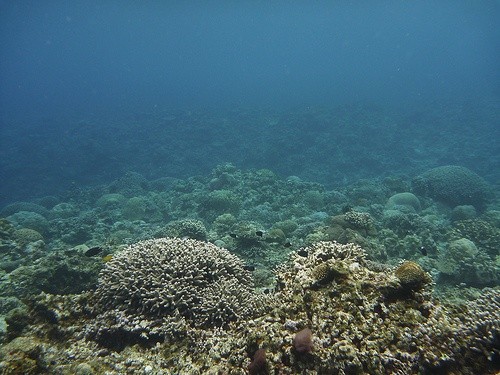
[418,245,427,256]
[84,247,103,257]
[290,327,314,355]
[246,348,267,374]
[229,231,308,294]
